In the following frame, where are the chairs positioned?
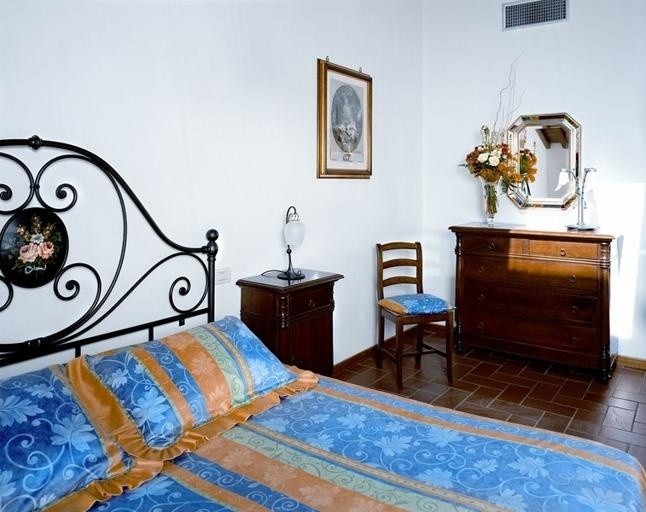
[375,241,455,391]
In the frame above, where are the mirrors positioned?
[503,111,582,210]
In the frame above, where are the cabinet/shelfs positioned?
[447,226,623,384]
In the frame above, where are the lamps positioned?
[277,205,307,281]
[554,167,601,231]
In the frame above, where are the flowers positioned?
[520,130,538,196]
[465,123,520,215]
[7,216,63,276]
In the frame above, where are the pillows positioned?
[0,364,164,512]
[377,293,457,315]
[62,315,320,463]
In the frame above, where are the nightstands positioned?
[235,268,345,380]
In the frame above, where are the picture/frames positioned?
[317,55,373,179]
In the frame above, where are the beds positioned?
[0,134,646,512]
